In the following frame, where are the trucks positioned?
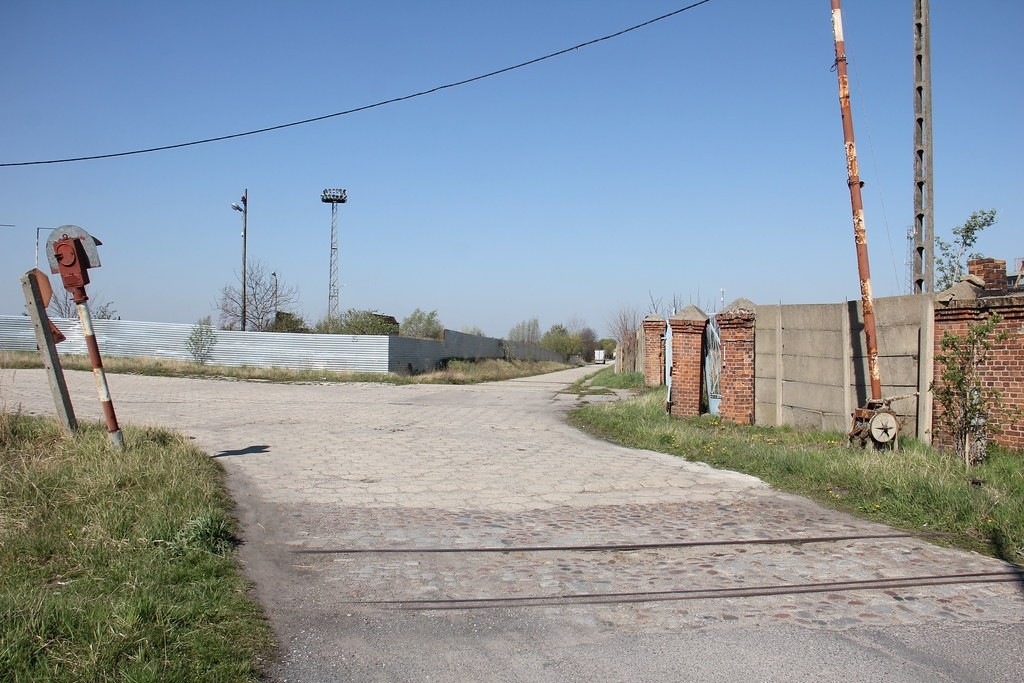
[594,350,606,364]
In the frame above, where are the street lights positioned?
[231,188,248,331]
[320,189,348,320]
[271,271,277,314]
